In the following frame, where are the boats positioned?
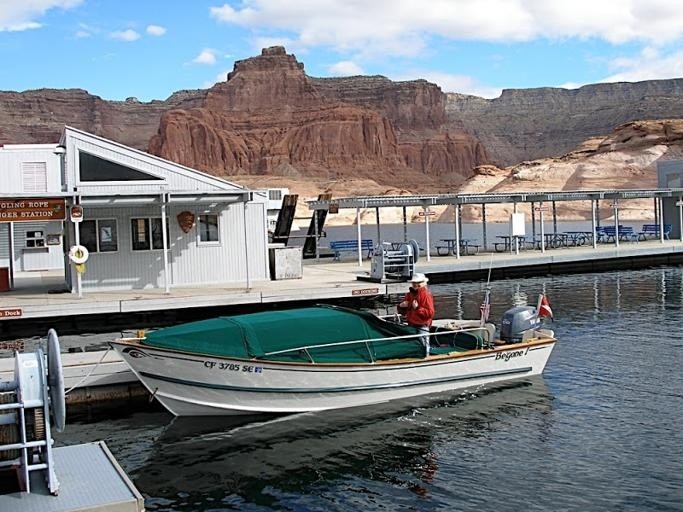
[106,303,558,414]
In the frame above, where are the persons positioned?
[399,273,434,357]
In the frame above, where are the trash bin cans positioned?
[0,267,9,292]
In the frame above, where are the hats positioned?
[407,273,429,283]
